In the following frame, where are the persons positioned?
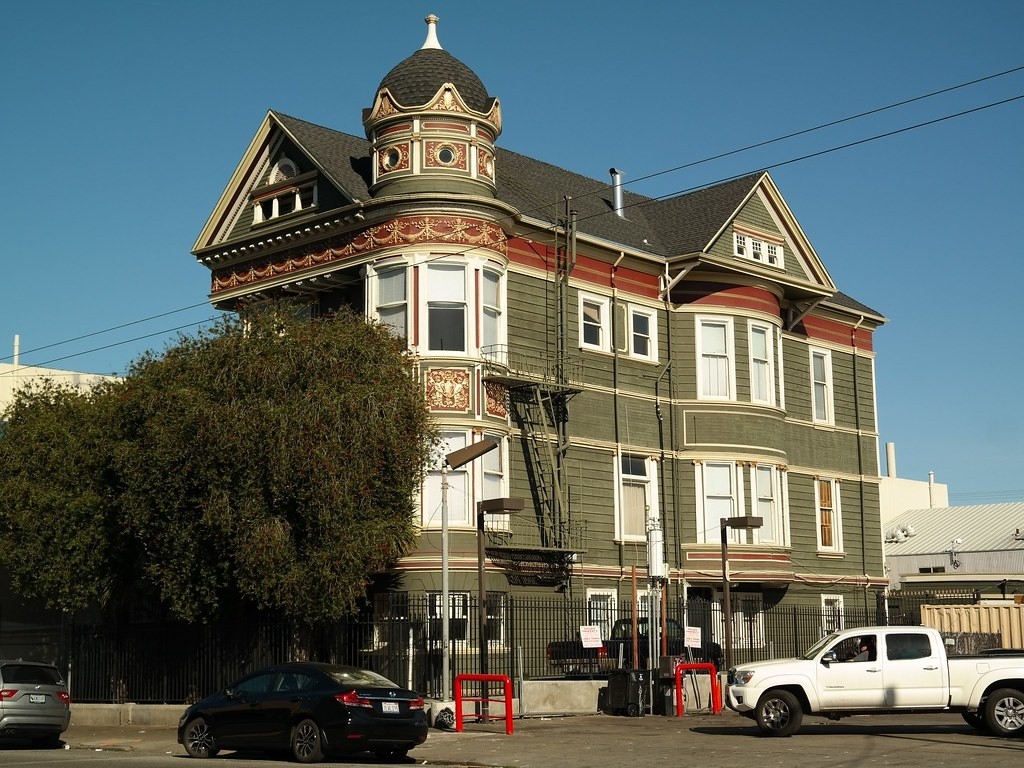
[846,641,869,661]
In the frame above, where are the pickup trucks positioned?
[725,626,1024,738]
[545,616,722,680]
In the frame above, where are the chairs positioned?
[266,674,298,692]
[13,666,33,684]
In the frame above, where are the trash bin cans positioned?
[607,668,653,717]
[372,641,427,696]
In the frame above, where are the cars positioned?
[177,660,429,763]
[0,660,70,743]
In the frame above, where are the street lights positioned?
[477,498,524,723]
[721,516,763,670]
[440,438,497,701]
